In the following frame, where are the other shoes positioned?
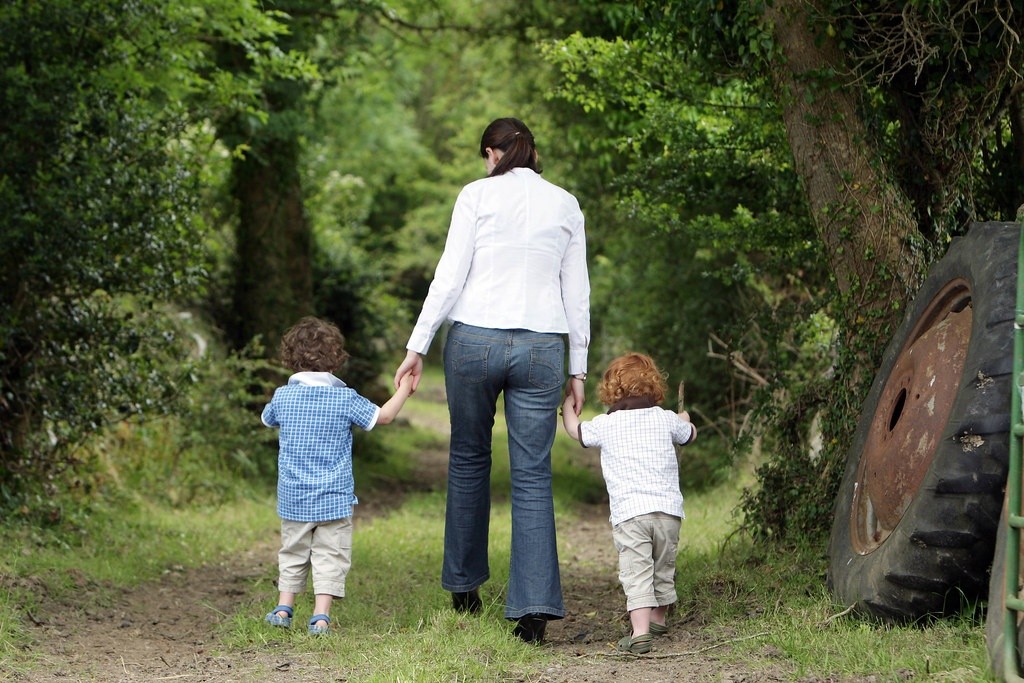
[510,614,547,646]
[451,588,482,618]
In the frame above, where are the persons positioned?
[563,353,697,654]
[261,316,415,635]
[397,118,590,643]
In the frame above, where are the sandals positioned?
[265,604,293,628]
[616,632,653,654]
[308,614,331,636]
[649,622,669,635]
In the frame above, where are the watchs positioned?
[570,374,587,383]
